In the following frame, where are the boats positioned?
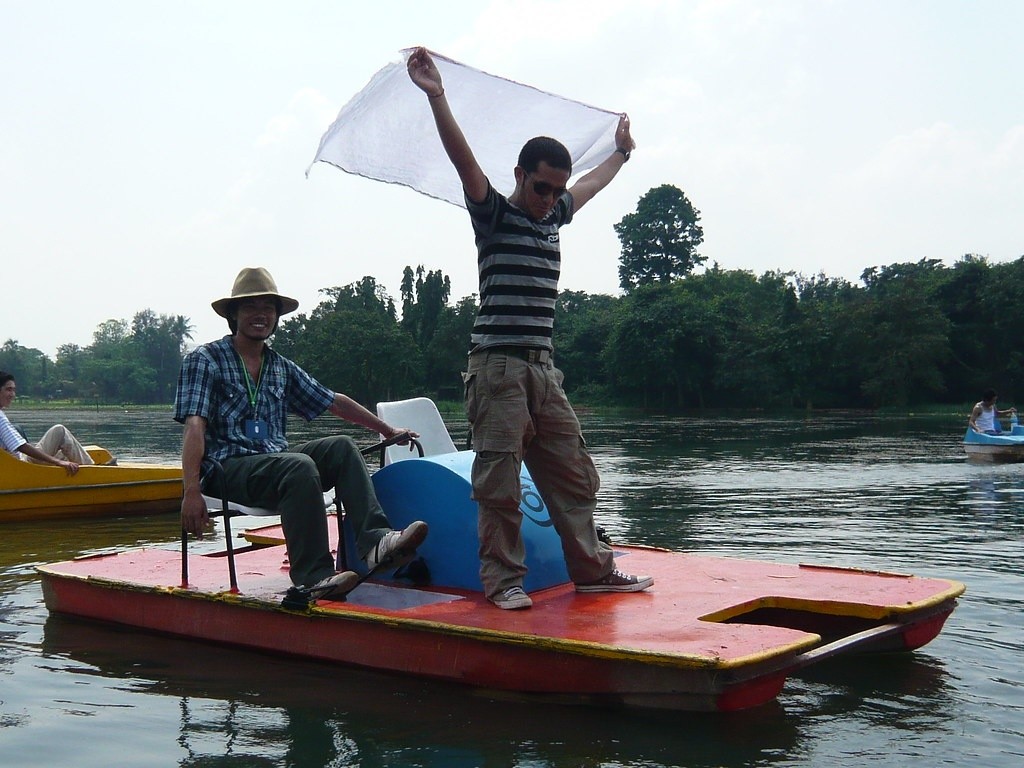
[956,412,1024,460]
[0,437,186,521]
[39,396,970,716]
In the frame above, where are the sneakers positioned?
[296,571,358,600]
[367,521,428,569]
[574,570,654,593]
[489,586,532,609]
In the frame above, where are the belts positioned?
[487,344,551,363]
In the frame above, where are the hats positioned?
[211,267,299,319]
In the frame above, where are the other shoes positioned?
[103,457,117,466]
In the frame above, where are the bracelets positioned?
[427,89,444,98]
[615,147,630,163]
[384,429,394,436]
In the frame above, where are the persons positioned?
[172,267,429,599]
[0,371,118,474]
[406,48,635,609]
[969,388,1017,436]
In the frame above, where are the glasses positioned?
[522,168,567,199]
